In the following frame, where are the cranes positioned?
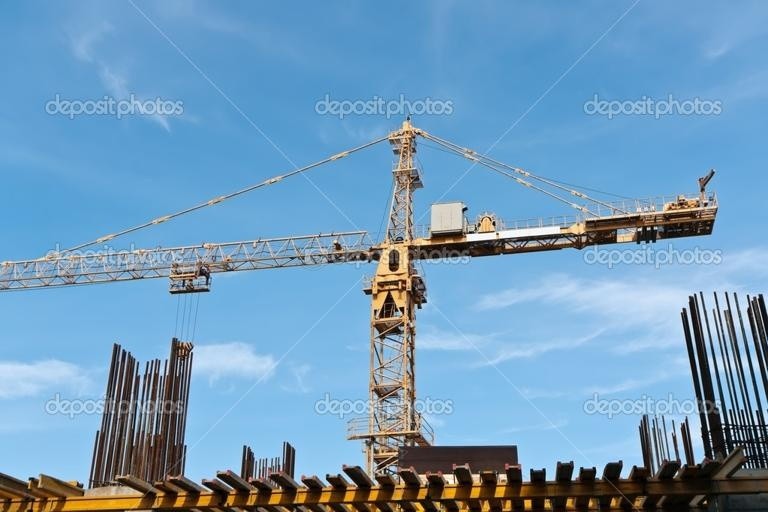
[0,114,717,483]
